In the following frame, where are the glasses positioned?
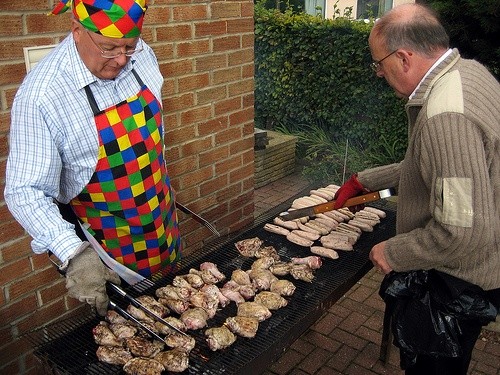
[369,49,412,73]
[86,31,144,59]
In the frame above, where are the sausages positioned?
[264,184,386,259]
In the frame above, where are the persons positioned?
[4,0,186,320]
[330,4,500,375]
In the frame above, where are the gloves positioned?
[334,174,370,213]
[65,248,121,316]
